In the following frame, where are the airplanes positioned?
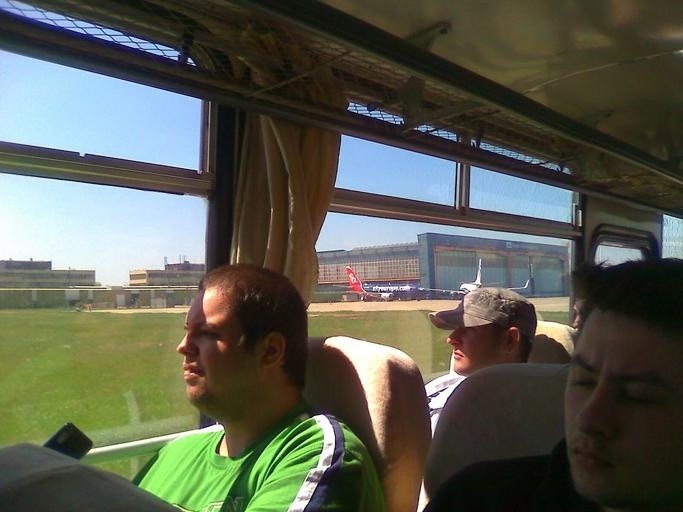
[415,258,529,299]
[332,264,429,302]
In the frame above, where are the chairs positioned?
[0,440,186,512]
[421,360,572,506]
[523,320,577,363]
[299,336,432,512]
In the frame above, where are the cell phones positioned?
[41,422,93,460]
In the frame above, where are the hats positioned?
[428,287,537,338]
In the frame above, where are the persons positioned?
[425,257,683,512]
[132,263,386,511]
[426,287,537,439]
